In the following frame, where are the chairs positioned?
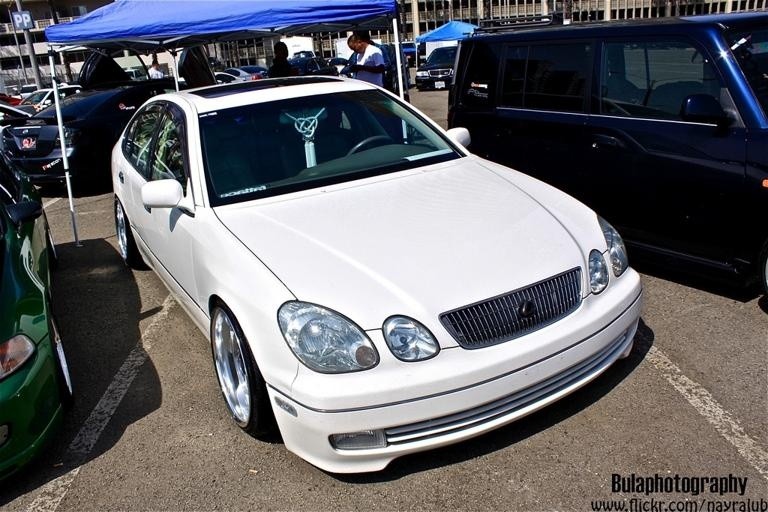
[201,102,353,194]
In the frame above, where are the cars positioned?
[292,51,348,74]
[2,83,153,201]
[108,73,647,480]
[0,148,75,482]
[215,63,267,85]
[414,46,459,91]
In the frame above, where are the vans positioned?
[447,12,765,306]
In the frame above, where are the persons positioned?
[342,35,385,86]
[359,32,391,73]
[266,42,297,79]
[146,60,165,80]
